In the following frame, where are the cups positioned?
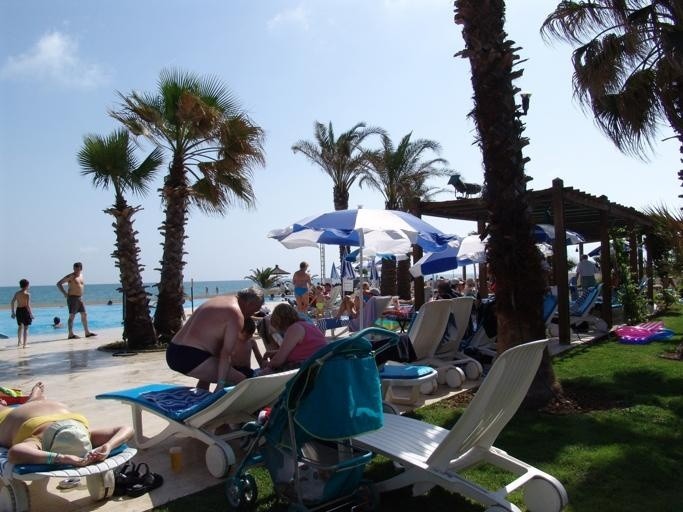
[169,446,184,473]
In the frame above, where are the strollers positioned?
[223,326,405,512]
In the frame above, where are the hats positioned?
[450,277,465,285]
[364,288,381,296]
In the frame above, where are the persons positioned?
[576,254,600,292]
[57,262,96,339]
[53,316,63,328]
[0,380,136,468]
[10,279,35,348]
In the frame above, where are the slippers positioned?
[68,333,97,339]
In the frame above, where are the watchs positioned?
[54,452,61,464]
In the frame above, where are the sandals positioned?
[112,462,135,497]
[127,462,164,498]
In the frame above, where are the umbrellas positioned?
[532,223,587,245]
[265,203,490,331]
[588,240,642,258]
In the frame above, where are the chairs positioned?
[341,337,569,511]
[1,398,138,510]
[247,277,653,408]
[95,369,301,478]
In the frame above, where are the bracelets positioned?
[11,311,14,313]
[47,451,52,465]
